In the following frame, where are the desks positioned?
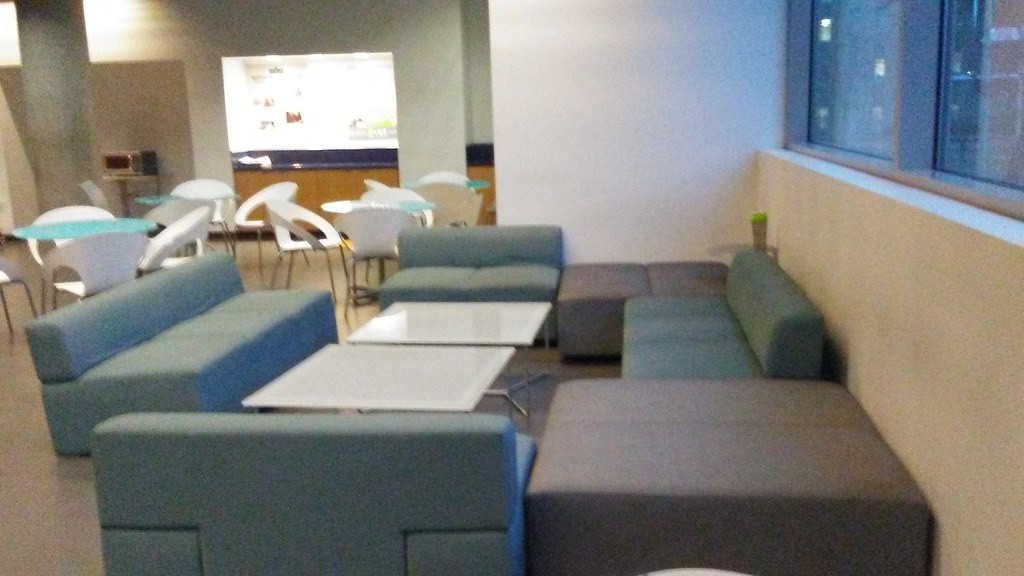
[10,217,155,309]
[395,199,433,228]
[242,342,517,416]
[463,179,489,195]
[138,196,187,209]
[346,299,552,413]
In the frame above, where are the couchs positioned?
[524,378,932,576]
[621,249,826,383]
[555,260,727,359]
[379,223,562,353]
[89,411,537,576]
[25,250,340,457]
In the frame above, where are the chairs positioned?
[138,207,210,278]
[415,171,476,196]
[0,260,39,335]
[364,177,390,195]
[233,181,303,272]
[415,181,484,230]
[170,178,237,261]
[339,203,420,304]
[361,188,436,229]
[40,231,152,313]
[265,195,350,304]
